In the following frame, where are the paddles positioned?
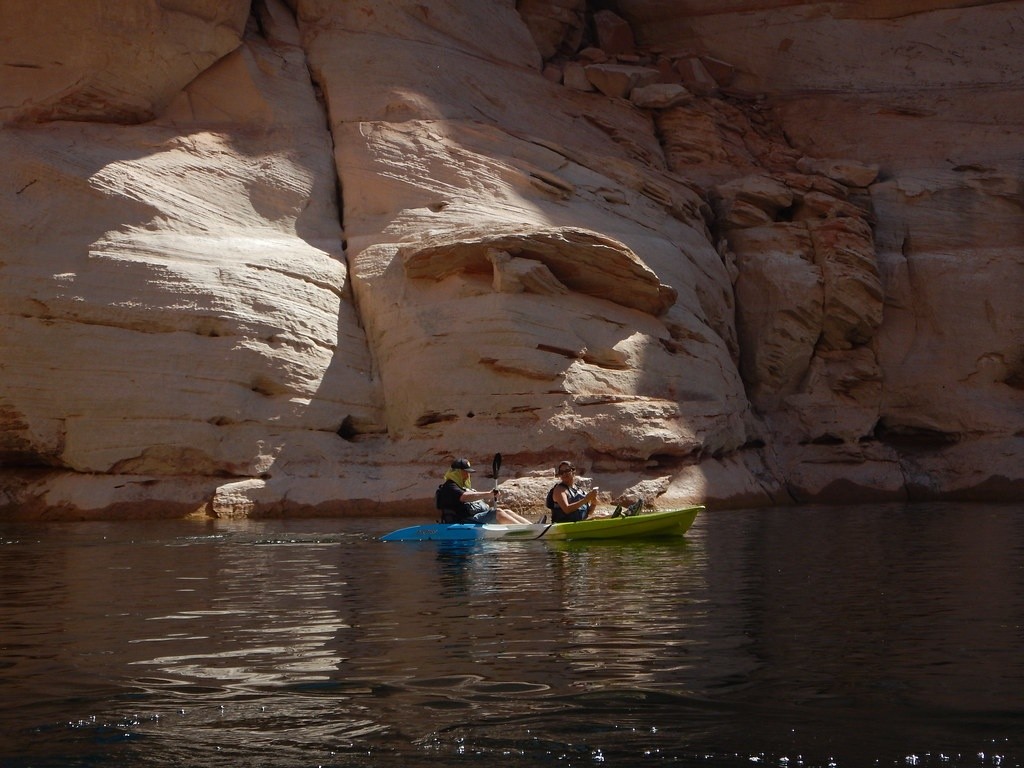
[492,452,502,508]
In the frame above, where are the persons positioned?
[551,461,642,523]
[439,458,547,524]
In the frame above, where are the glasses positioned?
[558,468,572,475]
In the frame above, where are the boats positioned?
[377,505,706,541]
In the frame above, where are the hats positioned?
[450,458,476,473]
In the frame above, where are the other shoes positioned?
[537,514,547,524]
[627,498,643,516]
